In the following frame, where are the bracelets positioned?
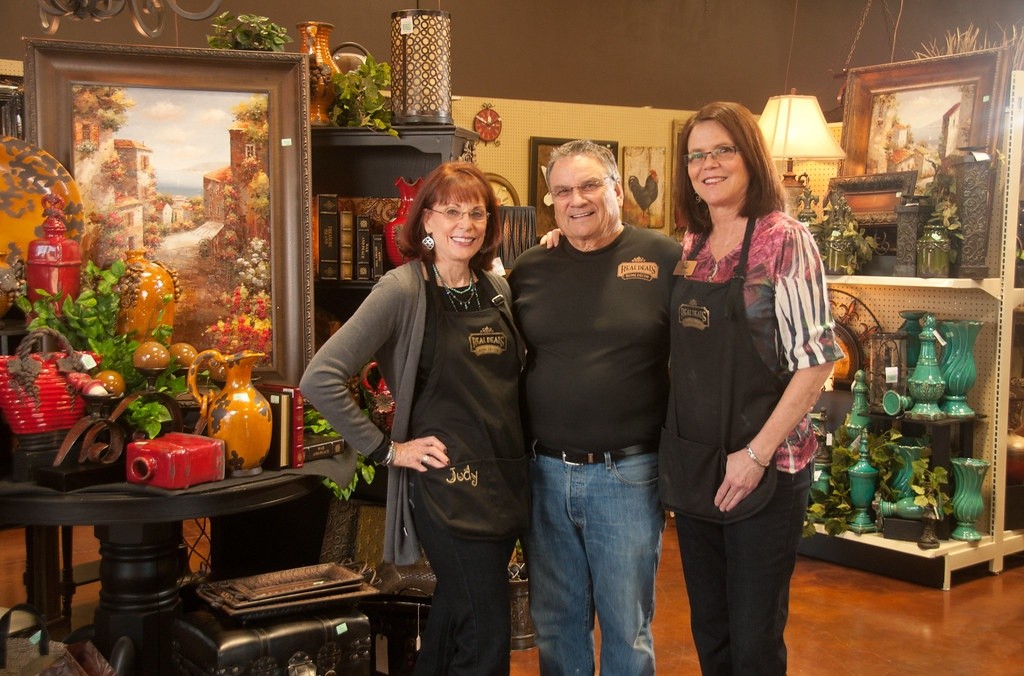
[382,441,394,464]
[746,444,771,467]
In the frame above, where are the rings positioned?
[423,456,430,463]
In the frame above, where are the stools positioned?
[145,606,371,676]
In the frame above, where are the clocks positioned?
[473,104,501,146]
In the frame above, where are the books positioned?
[318,192,384,281]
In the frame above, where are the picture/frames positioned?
[528,134,619,252]
[843,43,1013,190]
[20,35,320,396]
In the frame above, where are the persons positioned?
[505,139,685,676]
[299,161,528,676]
[539,99,844,676]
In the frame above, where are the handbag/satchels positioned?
[0,324,103,433]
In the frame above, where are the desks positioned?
[3,460,369,676]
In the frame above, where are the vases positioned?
[875,445,931,519]
[945,455,989,540]
[941,319,982,418]
[295,21,345,127]
[185,350,272,477]
[107,251,180,351]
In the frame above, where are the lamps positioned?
[756,89,847,215]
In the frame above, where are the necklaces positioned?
[433,263,481,312]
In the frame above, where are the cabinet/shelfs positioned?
[794,73,1024,593]
[311,128,471,320]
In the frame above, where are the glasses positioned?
[683,144,743,166]
[550,175,613,201]
[424,206,492,223]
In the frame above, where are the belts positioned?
[528,438,662,466]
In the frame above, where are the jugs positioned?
[187,349,273,477]
